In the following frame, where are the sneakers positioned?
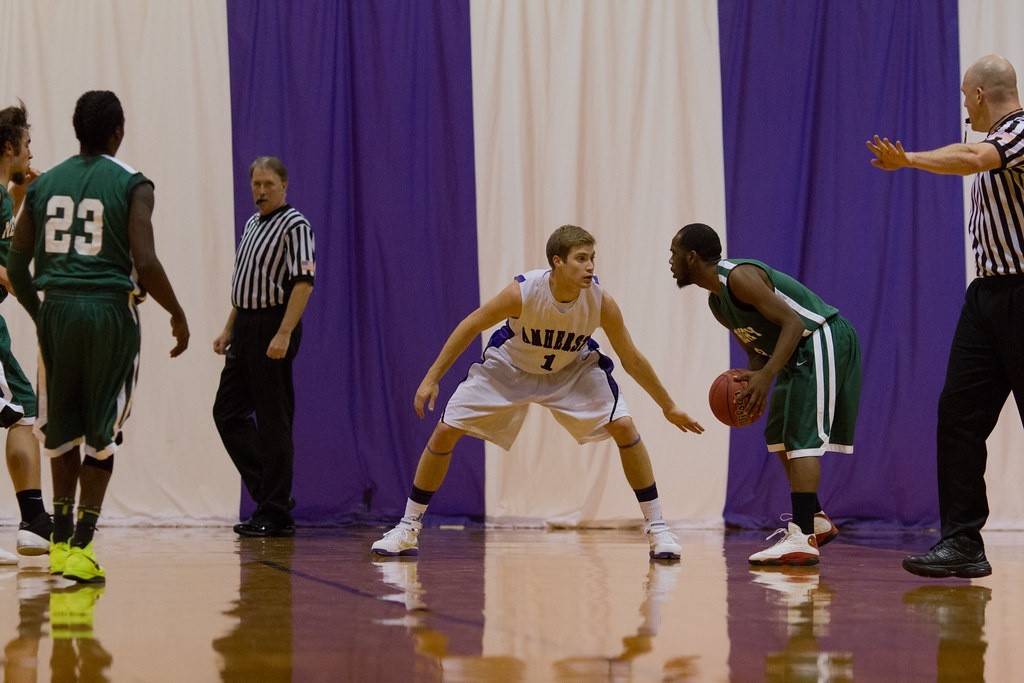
[62,537,106,583]
[0,547,19,565]
[640,521,683,560]
[233,508,296,538]
[903,535,993,579]
[49,533,68,576]
[17,513,54,556]
[779,511,838,549]
[371,519,422,555]
[748,521,820,566]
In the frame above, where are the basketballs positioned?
[708,367,765,429]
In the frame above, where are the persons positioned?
[1,105,52,562]
[370,223,705,557]
[864,54,1023,577]
[668,224,862,563]
[212,156,324,539]
[8,89,188,584]
